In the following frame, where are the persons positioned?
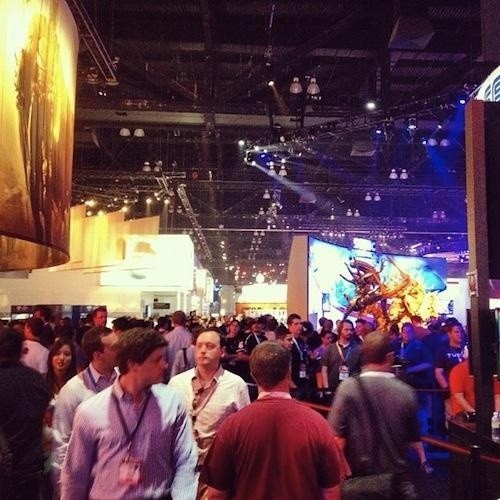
[1,302,500,500]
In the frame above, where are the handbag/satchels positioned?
[338,468,405,500]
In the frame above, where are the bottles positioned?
[491,412,500,441]
[321,291,331,312]
